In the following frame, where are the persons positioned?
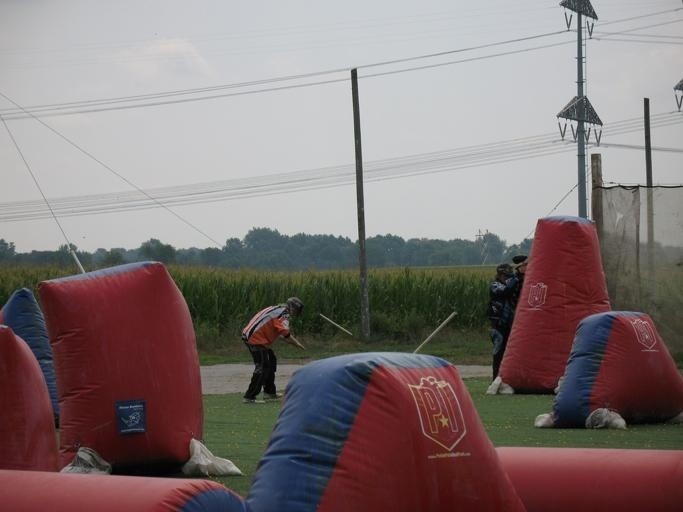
[497,252,528,337]
[239,295,307,405]
[486,262,514,381]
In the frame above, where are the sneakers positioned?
[241,391,283,405]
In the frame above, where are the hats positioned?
[287,296,304,310]
[496,255,529,274]
